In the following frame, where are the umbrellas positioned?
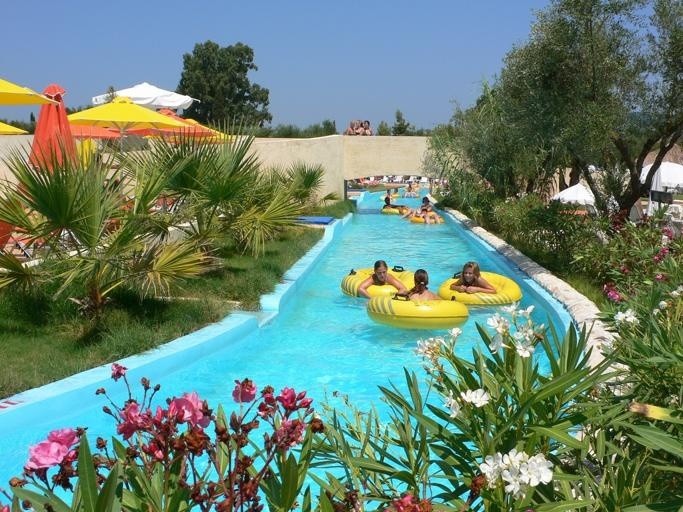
[646,165,663,217]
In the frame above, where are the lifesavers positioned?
[366,297,470,327]
[340,267,416,299]
[437,270,522,307]
[380,184,444,223]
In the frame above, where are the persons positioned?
[357,257,407,299]
[356,176,444,229]
[346,119,372,136]
[404,269,444,302]
[450,260,497,293]
